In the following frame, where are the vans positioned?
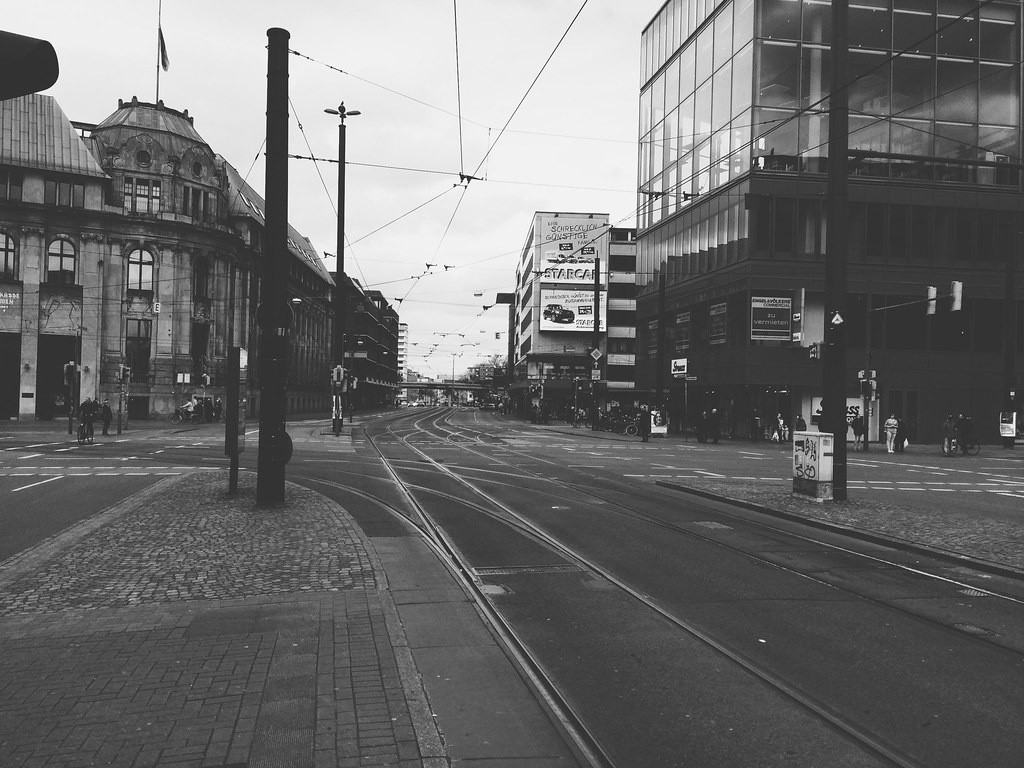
[445,398,453,406]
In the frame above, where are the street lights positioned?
[324,101,361,366]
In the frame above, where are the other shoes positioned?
[102,432,110,436]
[895,452,906,455]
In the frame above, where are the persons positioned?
[655,412,663,425]
[851,414,864,452]
[76,397,112,439]
[695,408,720,445]
[883,412,908,454]
[179,396,222,425]
[640,406,651,442]
[795,415,807,431]
[943,413,974,458]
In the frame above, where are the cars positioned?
[407,399,445,407]
[479,404,495,411]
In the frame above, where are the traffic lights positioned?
[950,280,962,312]
[330,364,357,393]
[926,285,937,314]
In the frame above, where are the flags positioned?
[159,27,170,72]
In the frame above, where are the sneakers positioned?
[888,451,894,454]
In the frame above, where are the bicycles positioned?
[170,407,199,425]
[77,415,94,445]
[942,428,981,455]
